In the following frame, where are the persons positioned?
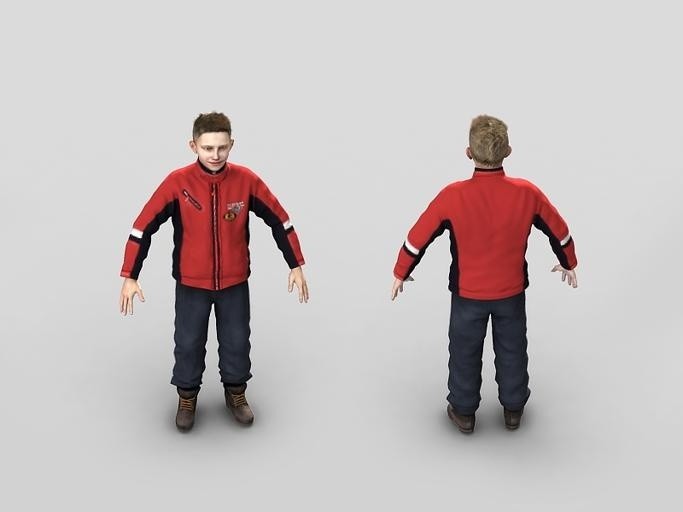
[390,114,577,433]
[119,112,309,433]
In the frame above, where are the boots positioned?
[447,403,475,435]
[504,403,524,430]
[174,388,199,430]
[223,386,255,425]
